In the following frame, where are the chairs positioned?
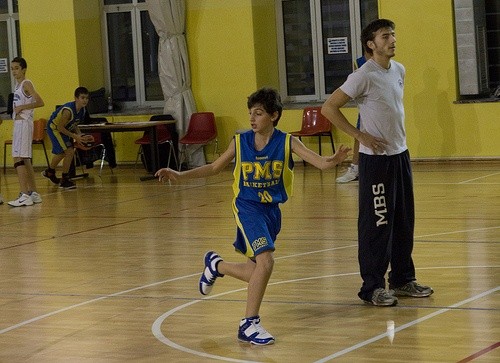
[70,128,106,178]
[3,120,49,171]
[287,106,335,167]
[135,114,180,176]
[178,112,217,165]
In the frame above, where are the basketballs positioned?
[77,134,95,151]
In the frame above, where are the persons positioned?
[6,57,45,206]
[154,89,352,344]
[336,27,372,183]
[320,18,433,308]
[42,87,89,189]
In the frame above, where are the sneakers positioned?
[199,251,224,295]
[364,287,399,306]
[237,315,275,346]
[390,280,434,297]
[336,163,359,183]
[42,170,60,184]
[7,192,34,206]
[59,179,77,187]
[28,191,42,203]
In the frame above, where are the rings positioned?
[375,145,377,148]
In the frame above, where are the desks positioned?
[45,121,173,173]
